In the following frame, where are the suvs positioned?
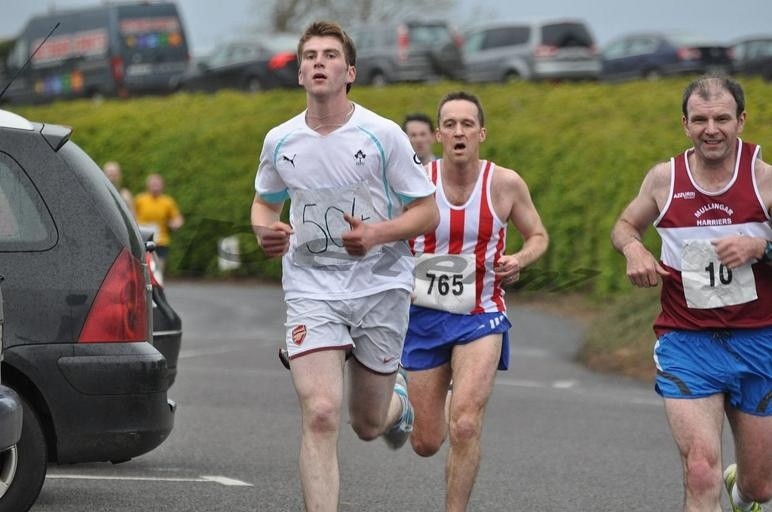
[0,20,176,512]
[347,19,459,86]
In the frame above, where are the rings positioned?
[508,276,513,281]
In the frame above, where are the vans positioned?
[457,19,606,89]
[0,2,189,103]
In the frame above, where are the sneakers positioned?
[382,373,415,450]
[723,465,764,512]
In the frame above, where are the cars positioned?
[133,225,182,392]
[726,36,772,85]
[180,29,306,100]
[598,31,731,82]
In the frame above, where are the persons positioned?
[250,23,440,511]
[609,73,772,511]
[402,115,436,166]
[400,92,550,511]
[134,173,185,289]
[101,161,135,218]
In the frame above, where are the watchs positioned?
[765,241,772,261]
